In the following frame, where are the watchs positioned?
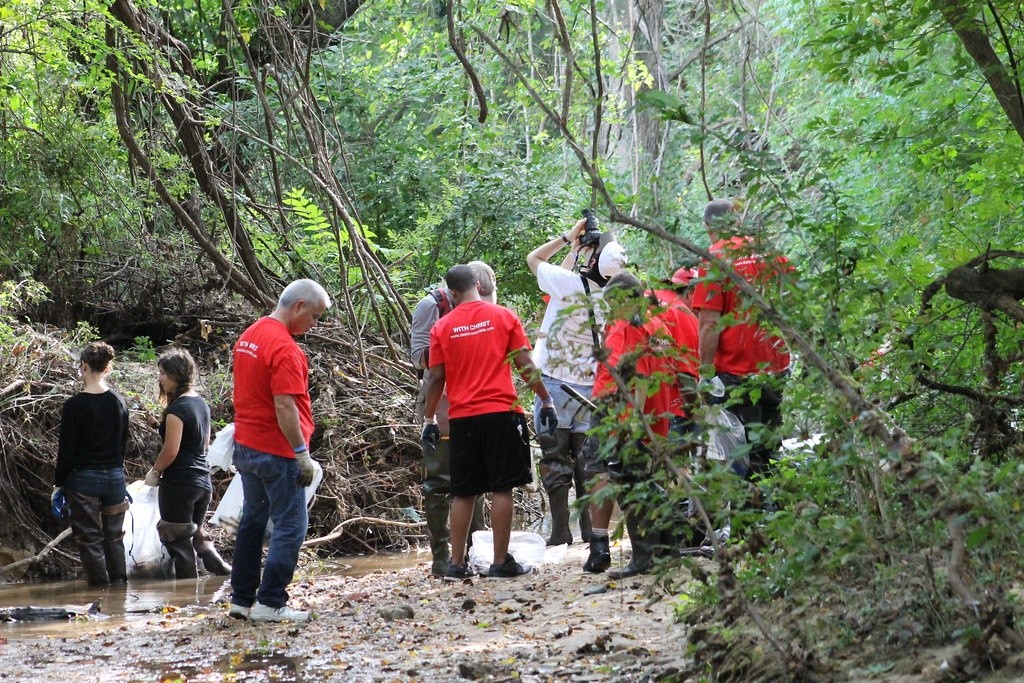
[151,467,160,476]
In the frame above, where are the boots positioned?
[609,443,680,579]
[535,428,593,545]
[72,491,127,587]
[420,439,485,574]
[157,520,232,580]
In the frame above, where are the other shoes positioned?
[584,536,611,572]
[701,543,725,559]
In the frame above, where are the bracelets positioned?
[295,444,307,453]
[562,234,571,246]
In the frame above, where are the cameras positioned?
[579,209,601,247]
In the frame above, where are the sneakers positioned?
[230,600,309,623]
[446,555,478,577]
[489,553,534,577]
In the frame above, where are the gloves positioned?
[421,415,441,454]
[295,450,314,487]
[145,468,161,487]
[697,376,726,406]
[540,398,559,436]
[50,488,72,522]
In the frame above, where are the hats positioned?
[704,198,737,227]
[466,261,497,304]
[659,267,698,287]
[597,230,628,282]
[602,271,643,299]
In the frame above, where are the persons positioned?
[230,279,334,623]
[144,351,231,577]
[53,342,133,590]
[410,199,796,581]
